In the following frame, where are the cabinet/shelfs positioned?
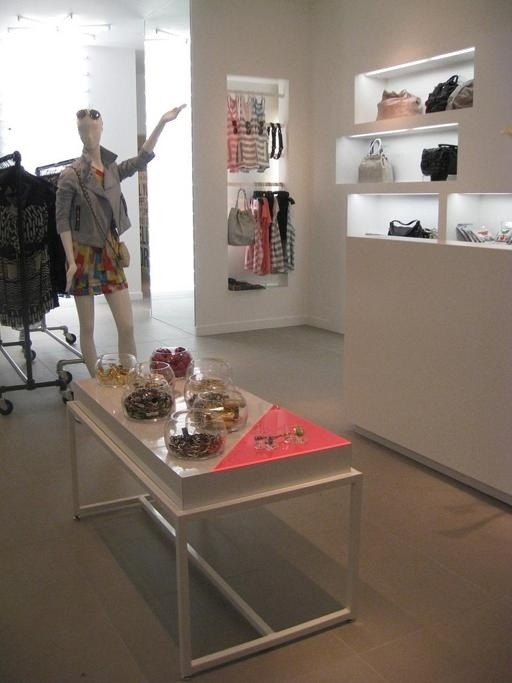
[333,45,512,251]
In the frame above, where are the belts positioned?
[268,122,283,158]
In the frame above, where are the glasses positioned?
[76,109,101,120]
[245,121,251,135]
[258,121,264,135]
[232,119,238,134]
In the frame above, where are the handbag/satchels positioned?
[426,76,473,113]
[377,90,423,120]
[359,152,393,182]
[228,208,255,245]
[388,221,439,239]
[118,242,130,267]
[421,144,457,174]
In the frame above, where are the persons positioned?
[54,100,190,377]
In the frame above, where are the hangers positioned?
[0,154,74,183]
[229,89,269,115]
[248,181,297,213]
